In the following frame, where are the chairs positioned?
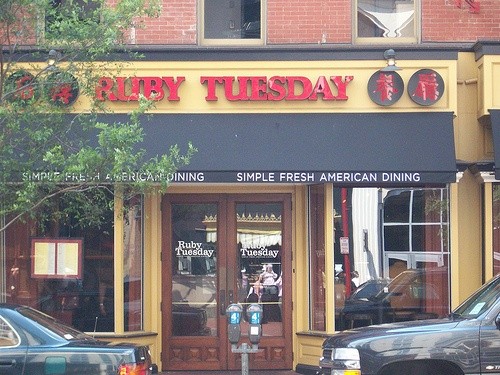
[334,269,420,327]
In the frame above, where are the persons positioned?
[257,264,277,285]
[275,271,282,297]
[240,269,251,292]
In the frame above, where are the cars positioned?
[0,300,156,375]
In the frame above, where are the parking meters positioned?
[226,303,263,374]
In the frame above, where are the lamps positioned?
[41,49,64,75]
[379,49,403,72]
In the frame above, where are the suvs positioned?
[317,274,500,375]
